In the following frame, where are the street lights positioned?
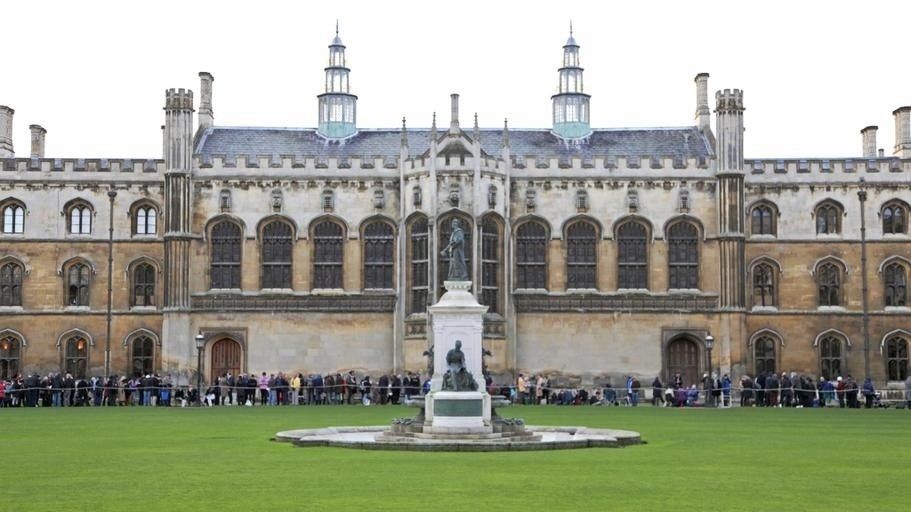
[194,331,205,407]
[705,332,714,406]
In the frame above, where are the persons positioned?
[441,219,468,281]
[446,341,468,391]
